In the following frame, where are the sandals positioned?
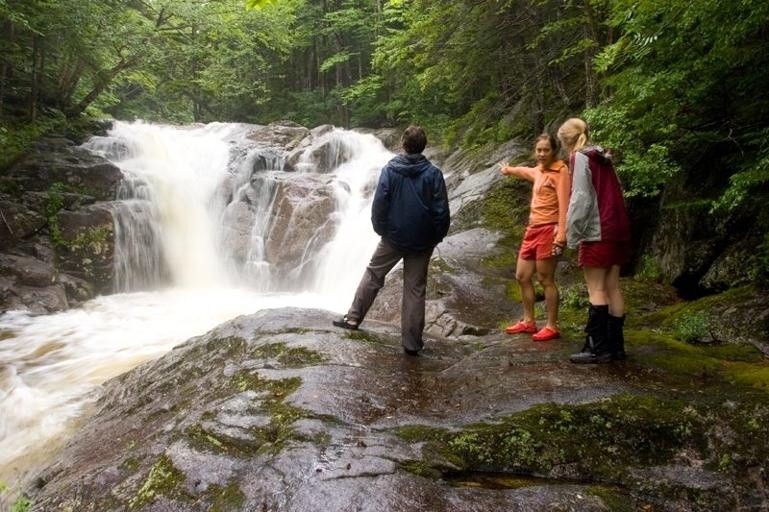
[405,349,417,356]
[532,326,560,341]
[505,319,537,333]
[333,319,358,330]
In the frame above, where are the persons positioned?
[332,126,450,356]
[556,116,630,365]
[496,132,572,342]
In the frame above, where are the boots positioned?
[569,302,626,363]
